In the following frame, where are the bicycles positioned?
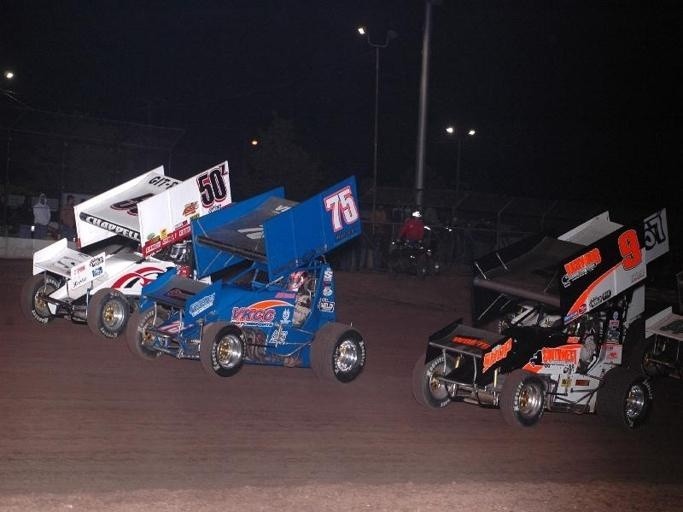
[391,240,428,280]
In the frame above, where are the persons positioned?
[31,192,51,239]
[0,193,15,238]
[394,209,426,274]
[55,194,75,241]
[15,197,35,239]
[370,202,394,270]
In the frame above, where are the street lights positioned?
[446,124,477,223]
[358,23,398,234]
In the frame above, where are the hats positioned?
[412,211,422,218]
